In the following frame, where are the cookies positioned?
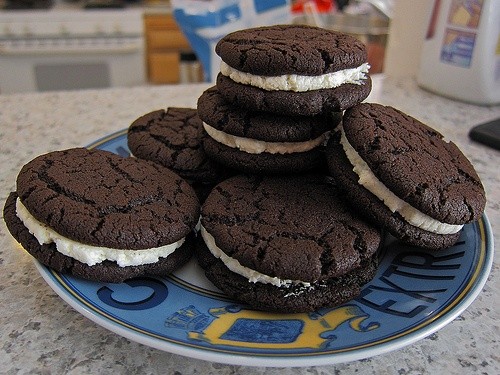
[3,22,484,315]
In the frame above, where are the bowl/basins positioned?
[291,15,391,75]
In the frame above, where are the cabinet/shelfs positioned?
[144,14,202,83]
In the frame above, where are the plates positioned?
[31,126,494,368]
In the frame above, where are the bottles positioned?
[415,0,500,107]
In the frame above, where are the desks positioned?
[0,74,500,375]
